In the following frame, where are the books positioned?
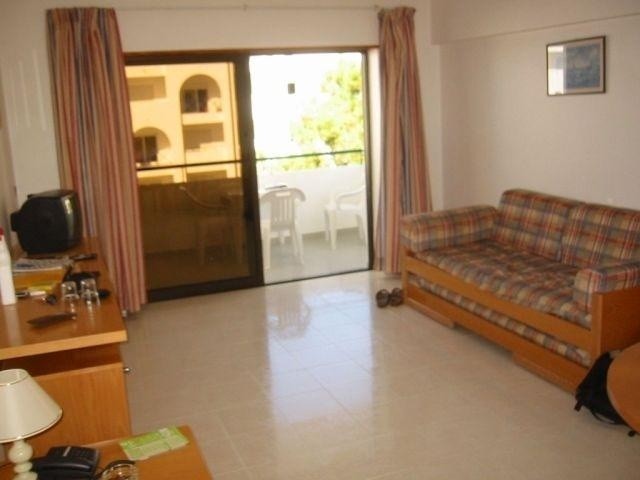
[12,258,74,298]
[118,425,189,462]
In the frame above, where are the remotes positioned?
[70,253,97,261]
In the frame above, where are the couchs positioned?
[398,187,640,397]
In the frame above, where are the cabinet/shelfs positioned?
[1,234,134,461]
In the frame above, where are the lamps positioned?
[0,368,63,480]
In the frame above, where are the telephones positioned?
[29,446,99,480]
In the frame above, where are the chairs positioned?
[177,167,369,270]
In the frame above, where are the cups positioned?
[59,279,100,304]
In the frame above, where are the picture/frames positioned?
[547,36,606,97]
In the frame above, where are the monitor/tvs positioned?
[11,189,83,256]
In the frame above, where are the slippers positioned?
[375,287,404,308]
